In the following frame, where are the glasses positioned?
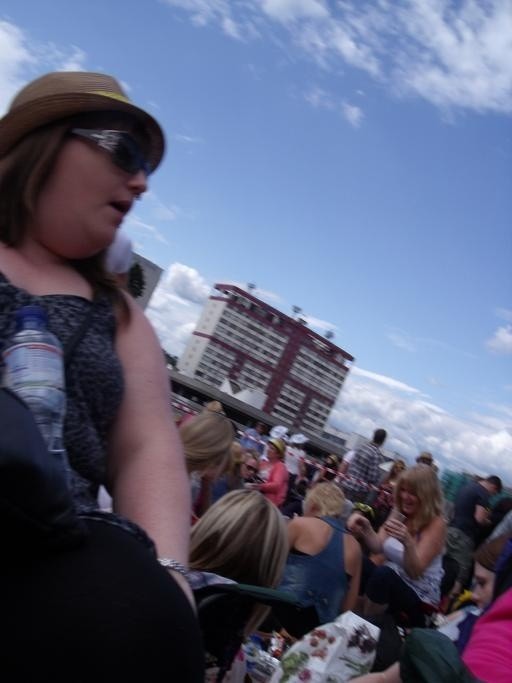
[393,465,405,471]
[242,462,259,474]
[67,126,157,175]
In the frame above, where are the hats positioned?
[289,433,310,446]
[268,436,287,456]
[414,451,434,462]
[0,68,167,178]
[229,441,246,465]
[201,399,226,417]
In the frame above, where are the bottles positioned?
[243,633,262,675]
[3,306,71,471]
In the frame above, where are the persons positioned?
[210,441,245,504]
[334,449,356,482]
[348,531,512,681]
[276,483,362,624]
[259,425,288,461]
[346,463,445,624]
[371,459,405,530]
[0,71,195,612]
[315,454,338,484]
[238,448,258,485]
[285,433,310,505]
[189,488,289,639]
[240,422,266,450]
[446,475,503,600]
[257,437,289,507]
[341,429,387,502]
[177,413,233,510]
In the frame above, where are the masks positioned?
[328,454,338,463]
